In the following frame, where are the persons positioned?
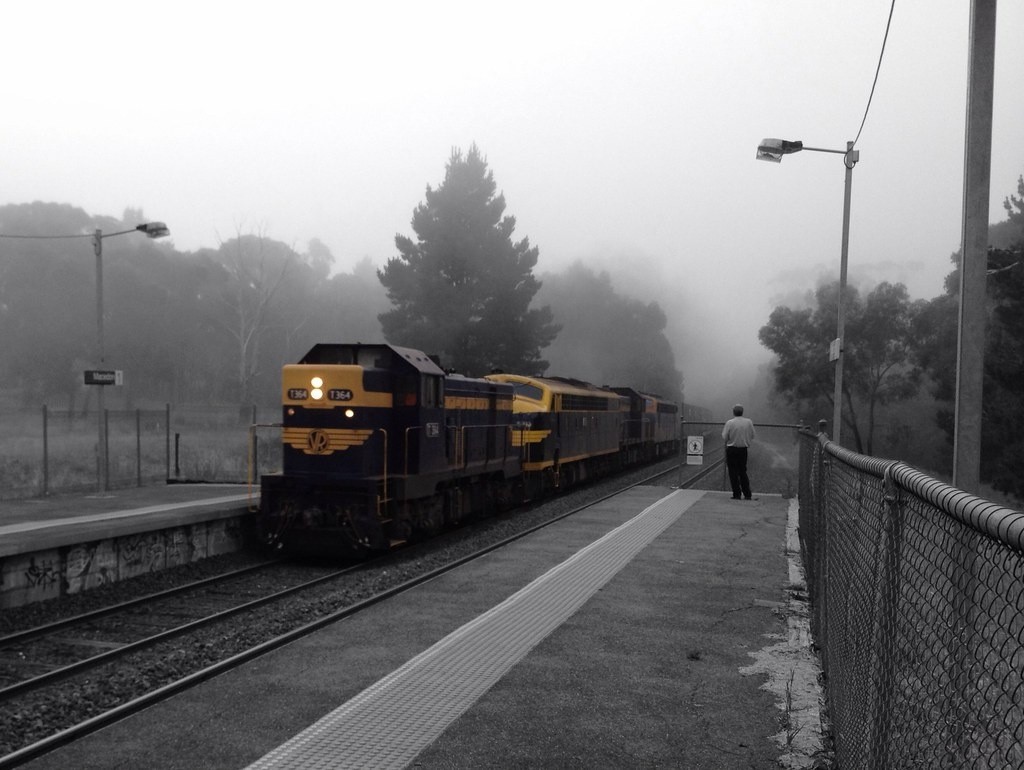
[721,404,755,501]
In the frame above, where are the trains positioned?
[259,341,715,552]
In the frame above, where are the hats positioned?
[732,404,742,411]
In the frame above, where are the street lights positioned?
[756,138,860,448]
[90,222,170,490]
